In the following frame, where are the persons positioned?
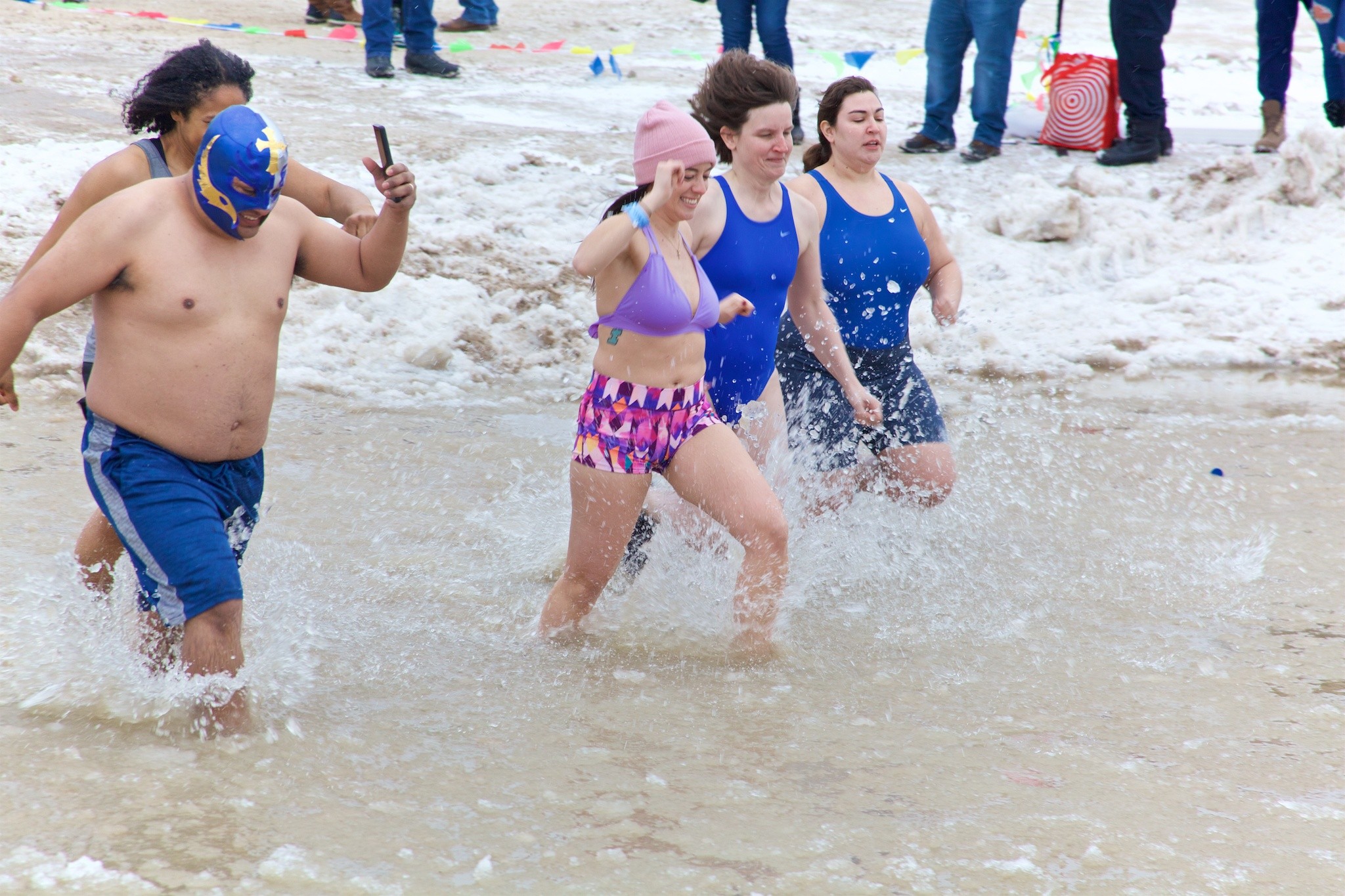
[10,40,378,595]
[1096,0,1177,166]
[533,101,790,635]
[782,76,960,524]
[0,106,416,728]
[306,0,499,78]
[623,48,885,574]
[1254,0,1345,153]
[716,0,804,146]
[896,0,1026,161]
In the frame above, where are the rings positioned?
[410,184,414,191]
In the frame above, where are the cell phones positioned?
[373,124,400,204]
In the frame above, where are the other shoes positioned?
[794,127,805,146]
[623,509,659,576]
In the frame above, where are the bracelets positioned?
[626,202,649,228]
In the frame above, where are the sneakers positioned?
[1255,99,1286,152]
[960,139,1001,158]
[365,56,396,79]
[440,16,491,32]
[306,0,328,22]
[406,51,459,77]
[1160,129,1176,156]
[1096,137,1160,166]
[899,131,944,154]
[327,1,363,26]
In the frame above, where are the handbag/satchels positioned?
[1040,54,1121,151]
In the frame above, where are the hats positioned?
[633,101,716,187]
